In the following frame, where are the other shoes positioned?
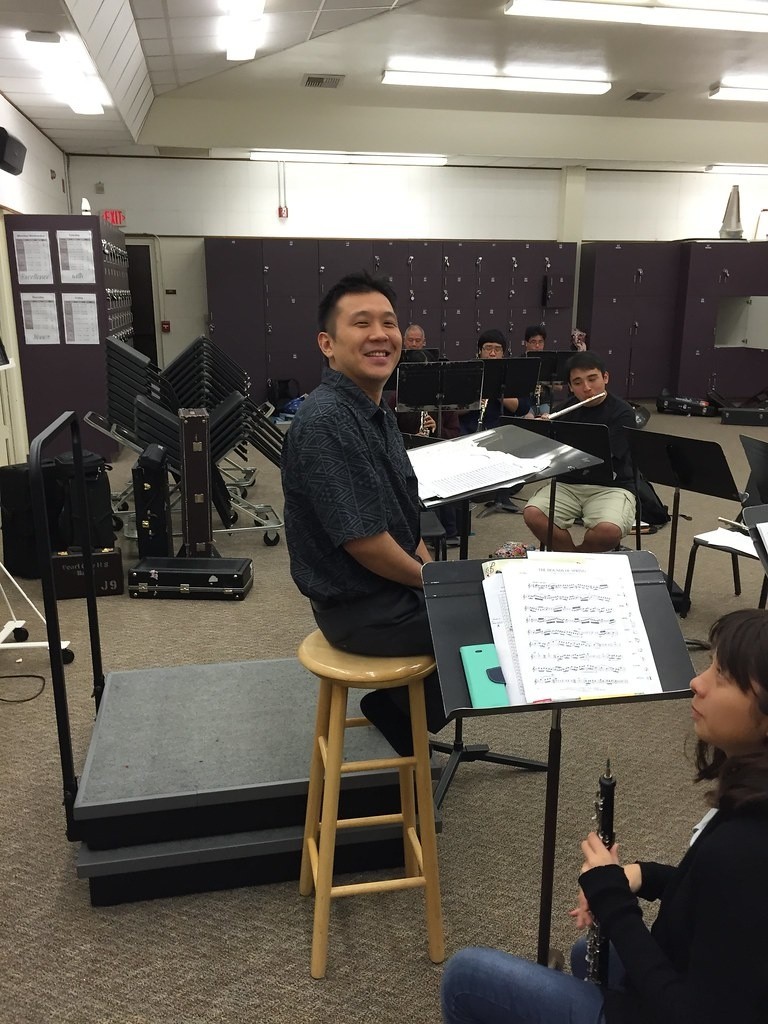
[360,686,432,758]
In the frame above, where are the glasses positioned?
[482,346,504,353]
[528,339,545,346]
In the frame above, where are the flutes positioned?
[418,411,430,437]
[585,756,618,983]
[535,391,607,420]
[476,398,490,431]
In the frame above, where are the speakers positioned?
[0,135,27,176]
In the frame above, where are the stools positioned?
[299,630,446,980]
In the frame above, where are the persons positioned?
[463,329,530,513]
[280,271,455,760]
[523,352,637,553]
[524,326,586,416]
[387,348,461,549]
[404,325,427,349]
[440,609,768,1024]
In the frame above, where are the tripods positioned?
[403,425,603,810]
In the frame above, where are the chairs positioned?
[685,475,768,614]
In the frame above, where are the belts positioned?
[310,598,339,612]
